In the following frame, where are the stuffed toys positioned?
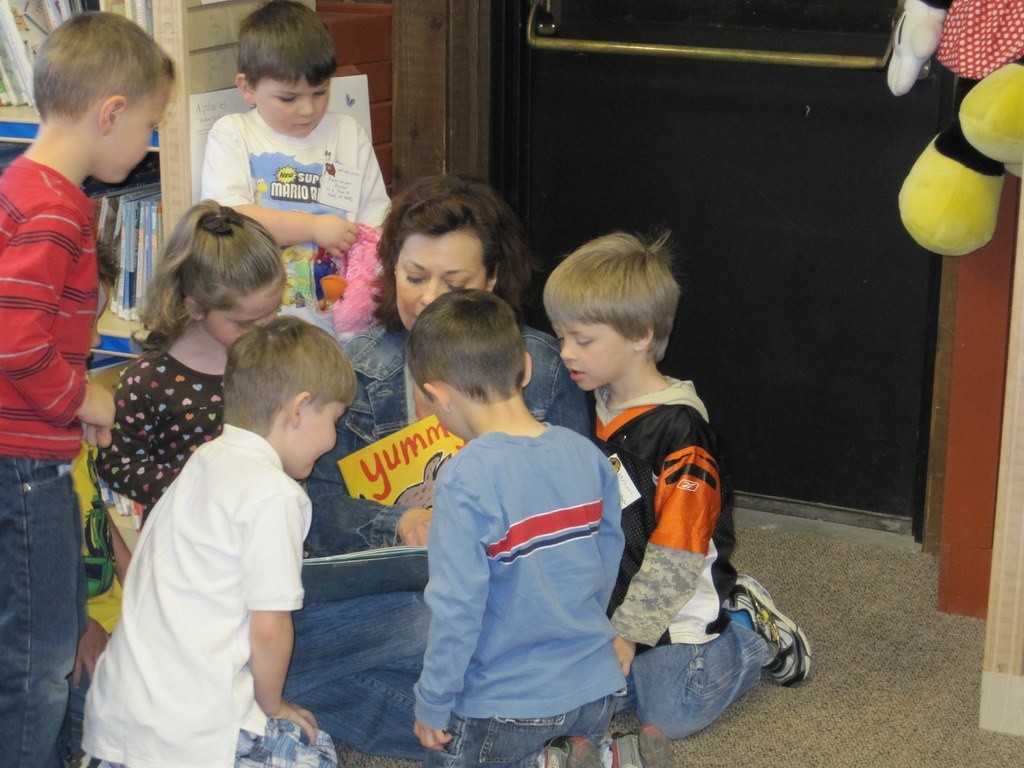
[885,1,1023,257]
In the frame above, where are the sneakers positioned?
[611,727,674,768]
[543,736,602,768]
[722,574,811,687]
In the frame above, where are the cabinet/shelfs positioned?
[0,0,320,360]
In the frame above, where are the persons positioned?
[80,315,357,768]
[203,1,391,346]
[541,232,814,738]
[406,288,673,767]
[0,14,176,768]
[74,171,593,767]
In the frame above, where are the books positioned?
[82,179,163,322]
[0,0,153,117]
